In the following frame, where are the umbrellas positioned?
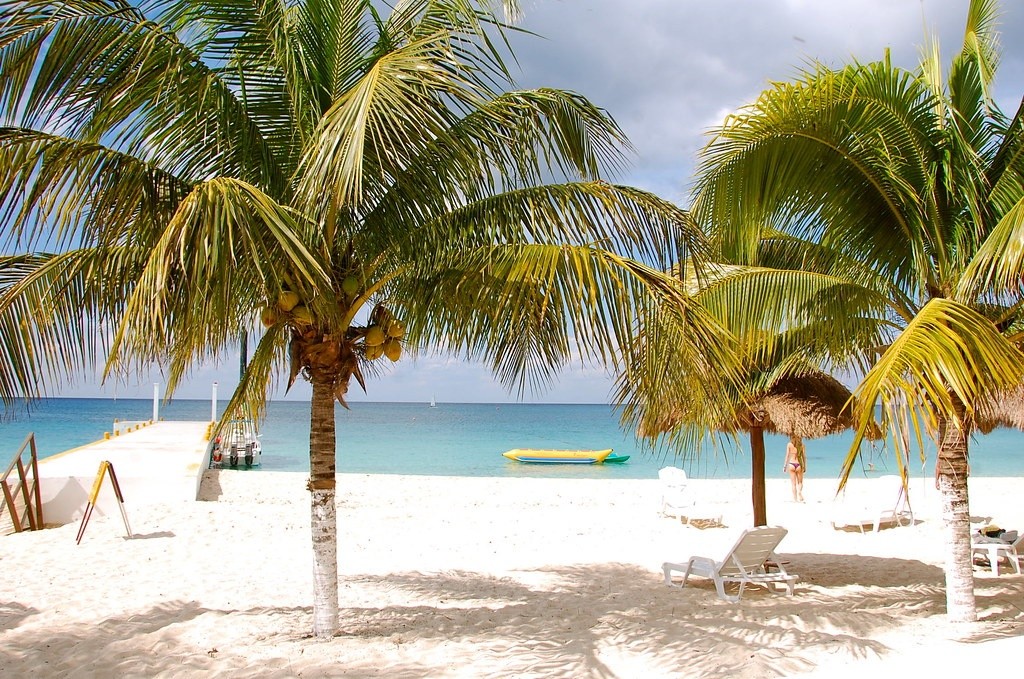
[965,302,1024,431]
[634,359,883,528]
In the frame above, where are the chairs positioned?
[661,524,799,603]
[658,465,725,528]
[971,511,1024,578]
[831,474,913,534]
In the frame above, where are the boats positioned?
[604,452,631,462]
[502,447,612,465]
[209,415,264,467]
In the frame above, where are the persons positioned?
[781,434,806,502]
